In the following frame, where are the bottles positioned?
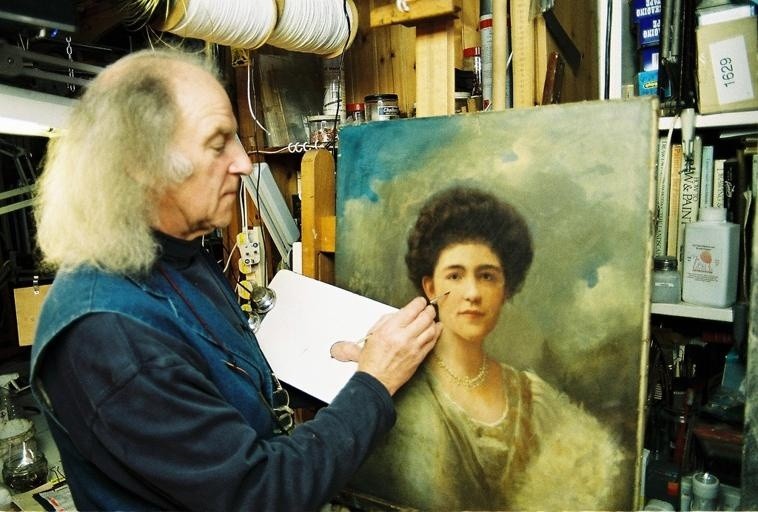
[682,207,743,305]
[652,254,682,304]
[0,420,49,496]
[363,94,400,124]
[460,47,484,96]
[308,115,342,145]
[346,103,366,124]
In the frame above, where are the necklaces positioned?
[431,351,490,389]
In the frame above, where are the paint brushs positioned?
[355,290,452,345]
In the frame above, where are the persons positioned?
[28,49,442,510]
[378,187,628,512]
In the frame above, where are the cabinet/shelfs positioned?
[596,0,758,322]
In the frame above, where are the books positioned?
[656,135,758,272]
